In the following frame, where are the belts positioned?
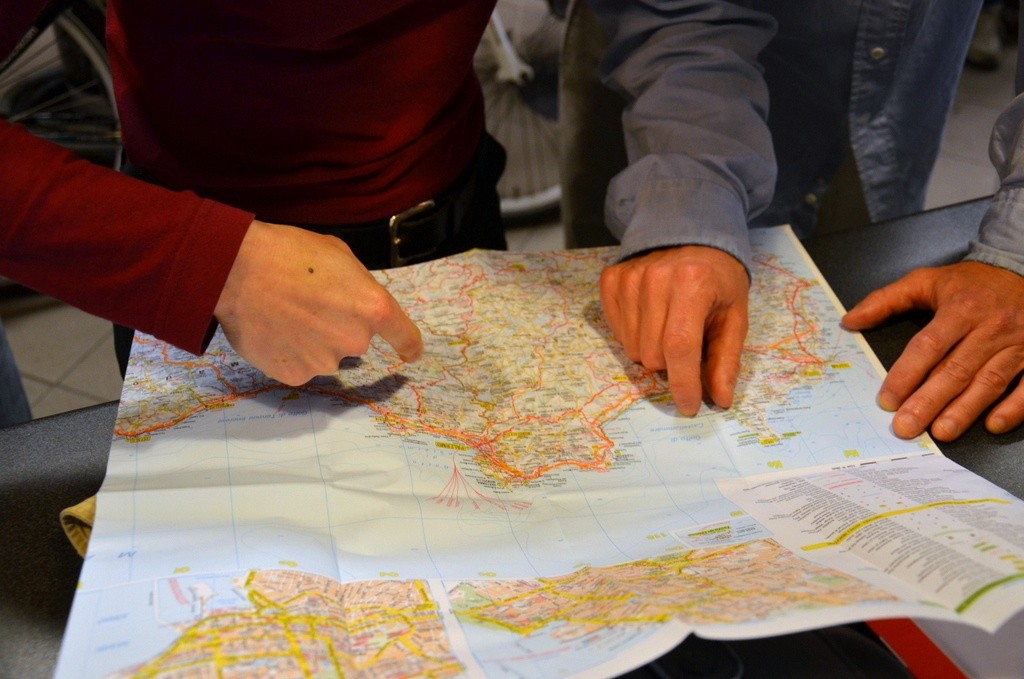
[294,165,483,268]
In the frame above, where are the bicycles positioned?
[0,0,571,301]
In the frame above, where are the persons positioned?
[599,0,1024,441]
[0,0,510,386]
